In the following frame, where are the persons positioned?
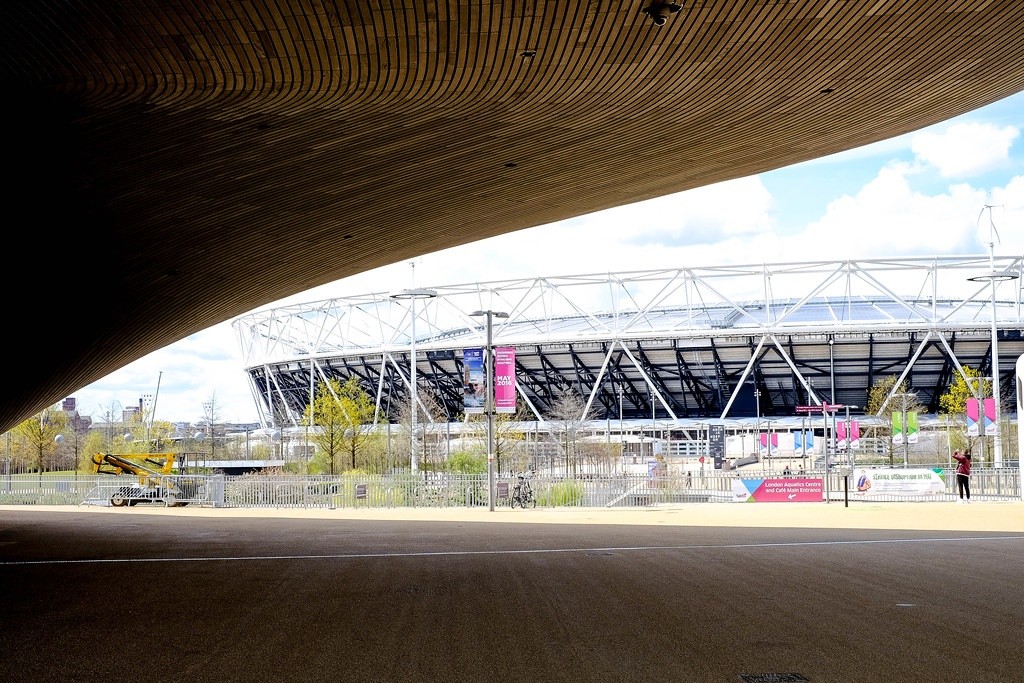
[686,471,692,489]
[783,465,792,479]
[952,449,971,504]
[796,464,806,478]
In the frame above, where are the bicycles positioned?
[509,472,538,509]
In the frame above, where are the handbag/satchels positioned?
[956,461,960,469]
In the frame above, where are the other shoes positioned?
[967,499,971,503]
[956,498,963,503]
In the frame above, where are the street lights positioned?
[468,309,509,512]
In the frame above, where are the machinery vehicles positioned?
[90,450,203,509]
